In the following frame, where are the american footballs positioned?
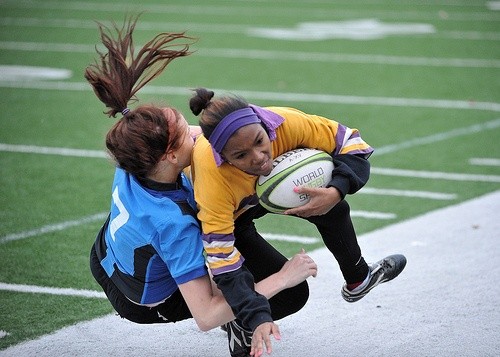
[255,147,337,214]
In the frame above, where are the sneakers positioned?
[227,320,253,357]
[343,255,407,304]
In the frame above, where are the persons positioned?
[188,86,407,357]
[83,7,319,332]
